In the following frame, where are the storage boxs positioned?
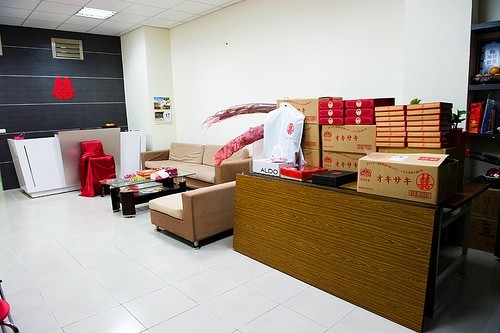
[277,96,459,205]
[467,187,500,253]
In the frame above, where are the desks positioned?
[250,173,489,320]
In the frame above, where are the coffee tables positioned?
[100,168,196,218]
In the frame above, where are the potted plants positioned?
[452,110,469,145]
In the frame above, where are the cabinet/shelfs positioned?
[463,0,500,255]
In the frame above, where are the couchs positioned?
[140,143,253,190]
[148,181,236,250]
